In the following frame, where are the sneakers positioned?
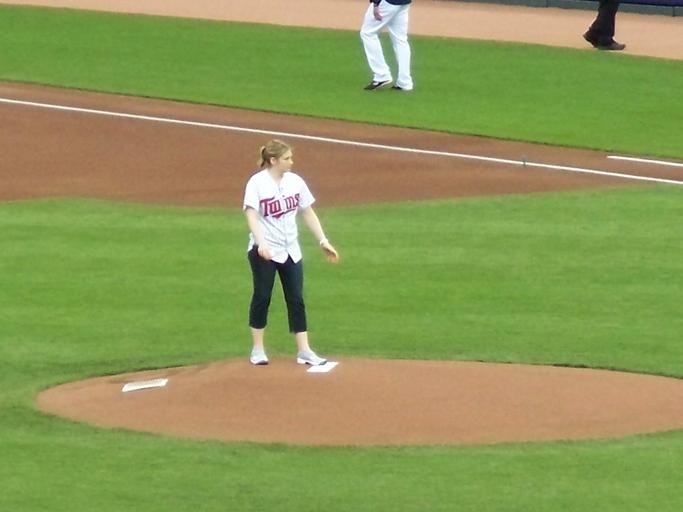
[297,351,327,365]
[584,31,625,51]
[393,80,412,90]
[250,349,267,365]
[364,79,391,90]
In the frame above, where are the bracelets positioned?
[319,237,330,247]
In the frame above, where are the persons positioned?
[357,0,419,94]
[239,138,342,366]
[577,0,628,51]
